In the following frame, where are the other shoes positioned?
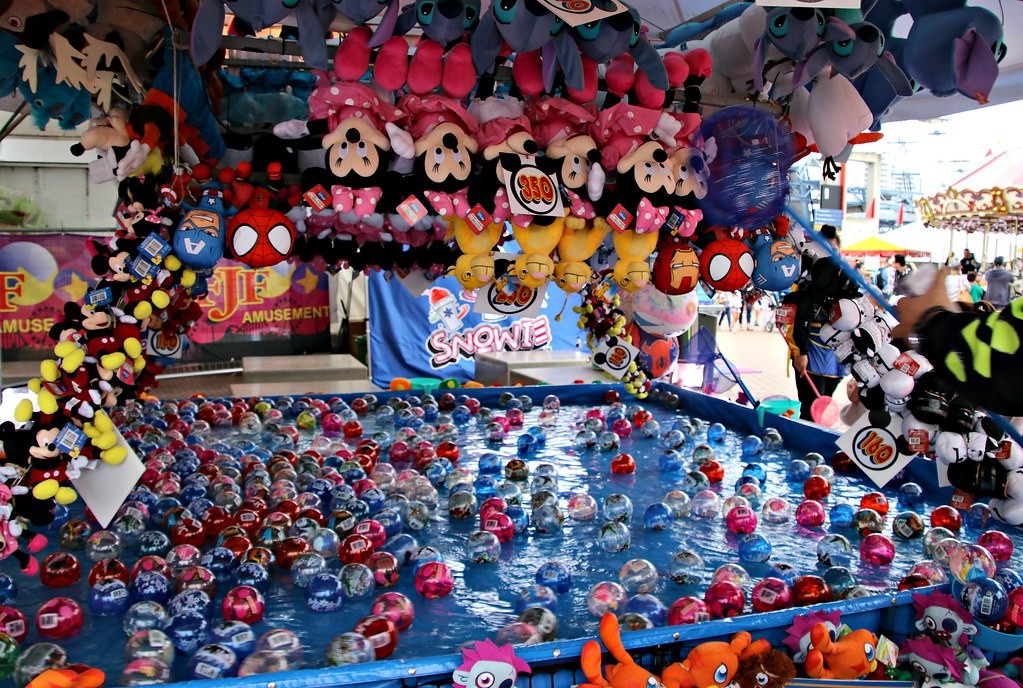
[747,328,753,331]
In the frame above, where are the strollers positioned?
[765,290,790,332]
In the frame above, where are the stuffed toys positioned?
[0,0,1011,290]
[452,587,1023,687]
[1,240,204,576]
[784,259,1022,526]
[576,281,652,399]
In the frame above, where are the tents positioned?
[838,236,933,263]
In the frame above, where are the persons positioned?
[777,226,843,423]
[719,291,764,334]
[852,253,913,306]
[943,248,1015,311]
